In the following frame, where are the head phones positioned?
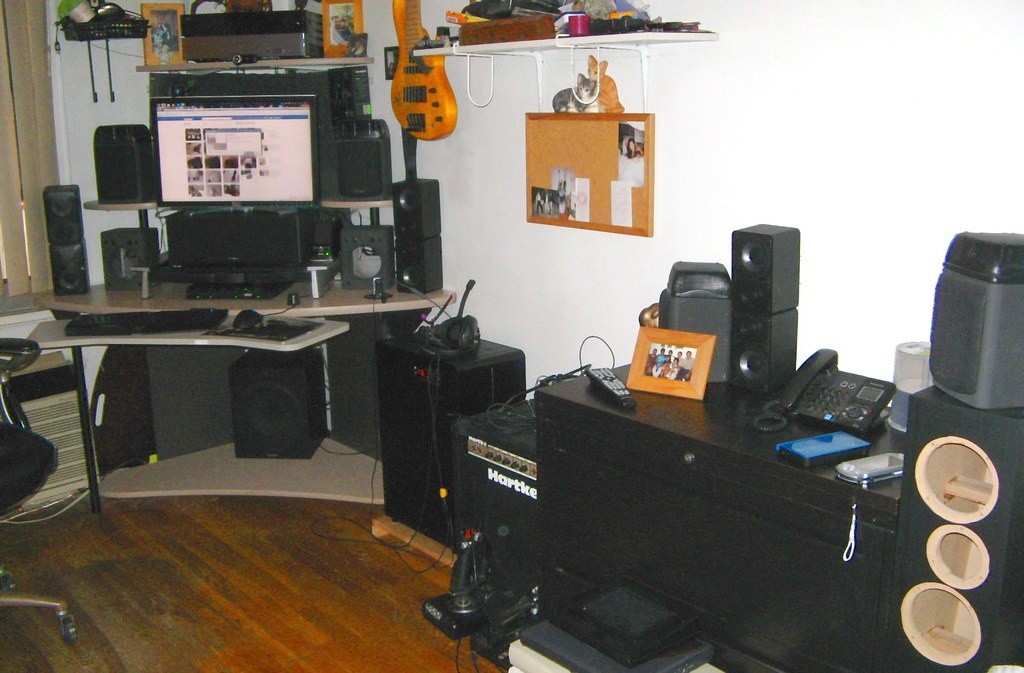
[412,316,480,358]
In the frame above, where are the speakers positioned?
[339,225,392,288]
[93,124,157,204]
[375,334,527,547]
[395,236,443,295]
[100,227,160,291]
[658,261,732,381]
[43,185,84,244]
[448,399,542,600]
[928,232,1024,410]
[227,345,328,459]
[49,239,90,297]
[391,177,441,241]
[730,225,799,314]
[729,308,799,392]
[335,117,392,201]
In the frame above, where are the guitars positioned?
[389,1,457,142]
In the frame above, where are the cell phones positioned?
[834,452,905,484]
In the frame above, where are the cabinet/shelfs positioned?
[528,362,910,673]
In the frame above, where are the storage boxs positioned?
[180,10,324,64]
[459,15,556,46]
[61,20,149,41]
[554,11,586,31]
[437,26,460,38]
[609,10,638,20]
[519,617,716,673]
[548,576,699,669]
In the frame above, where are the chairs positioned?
[0,337,79,644]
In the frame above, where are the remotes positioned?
[585,368,637,411]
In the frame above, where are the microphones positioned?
[456,279,476,320]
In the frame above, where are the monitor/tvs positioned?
[148,94,319,208]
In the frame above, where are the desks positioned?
[35,283,459,516]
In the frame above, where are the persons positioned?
[535,191,552,216]
[625,138,644,160]
[556,170,572,214]
[151,16,178,60]
[332,15,354,47]
[645,348,695,382]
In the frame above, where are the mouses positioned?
[233,309,263,329]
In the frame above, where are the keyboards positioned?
[63,307,229,337]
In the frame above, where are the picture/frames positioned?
[384,46,399,80]
[140,3,186,65]
[625,325,718,401]
[321,0,364,58]
[349,33,368,57]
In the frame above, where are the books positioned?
[508,574,727,673]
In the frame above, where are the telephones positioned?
[771,348,896,440]
